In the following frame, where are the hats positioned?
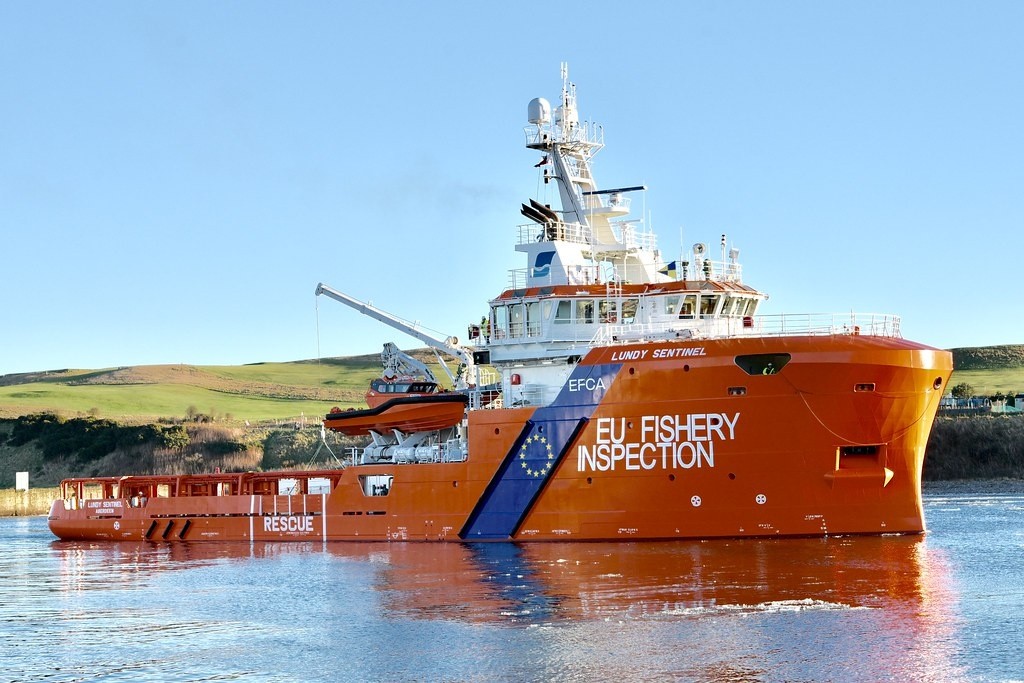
[139,492,143,495]
[481,316,486,320]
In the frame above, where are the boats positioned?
[47,62,954,541]
[325,377,467,436]
